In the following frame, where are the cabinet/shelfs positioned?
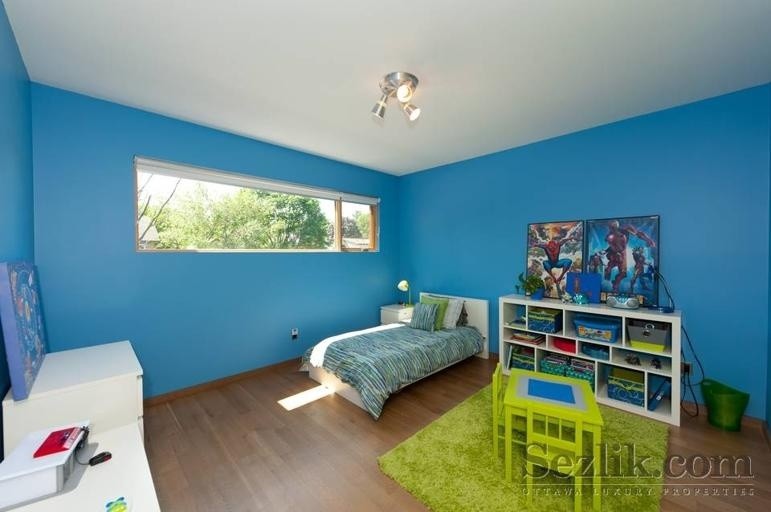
[497,293,683,428]
[0,339,144,446]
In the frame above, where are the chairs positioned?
[524,407,584,512]
[492,363,526,459]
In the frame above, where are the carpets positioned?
[378,374,671,512]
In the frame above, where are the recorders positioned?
[608,293,640,311]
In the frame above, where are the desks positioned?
[504,366,604,512]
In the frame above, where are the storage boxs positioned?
[511,308,670,407]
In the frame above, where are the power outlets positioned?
[681,361,694,375]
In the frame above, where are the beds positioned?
[299,291,490,421]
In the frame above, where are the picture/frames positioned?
[526,220,584,298]
[585,216,660,307]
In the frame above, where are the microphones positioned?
[648,265,675,312]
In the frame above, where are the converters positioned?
[684,365,689,372]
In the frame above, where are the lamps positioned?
[398,281,416,307]
[373,71,422,123]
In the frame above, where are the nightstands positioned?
[379,304,415,325]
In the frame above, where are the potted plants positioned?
[514,271,545,300]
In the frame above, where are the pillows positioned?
[411,295,468,332]
